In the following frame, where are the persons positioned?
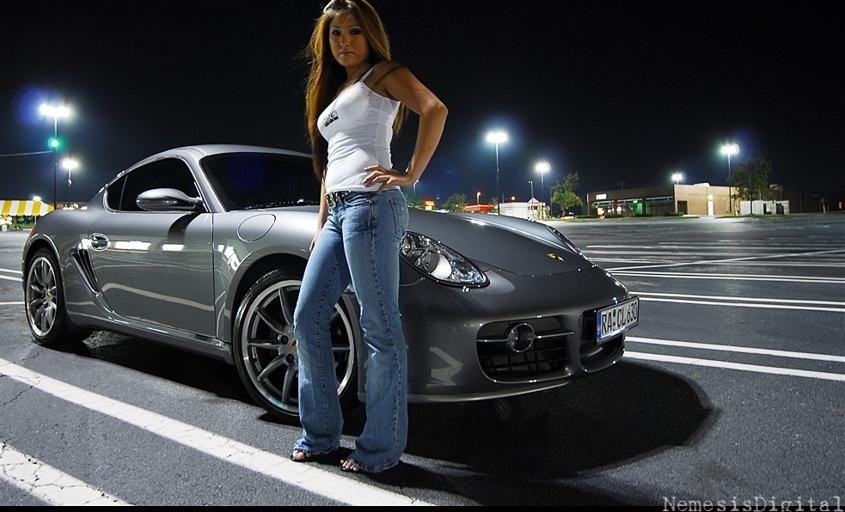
[291,0,450,476]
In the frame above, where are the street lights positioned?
[724,144,737,212]
[39,103,70,209]
[59,157,78,205]
[537,162,547,220]
[488,132,506,215]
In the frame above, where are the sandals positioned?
[288,442,342,462]
[339,455,366,473]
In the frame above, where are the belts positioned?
[324,190,353,207]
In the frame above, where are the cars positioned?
[22,144,639,425]
